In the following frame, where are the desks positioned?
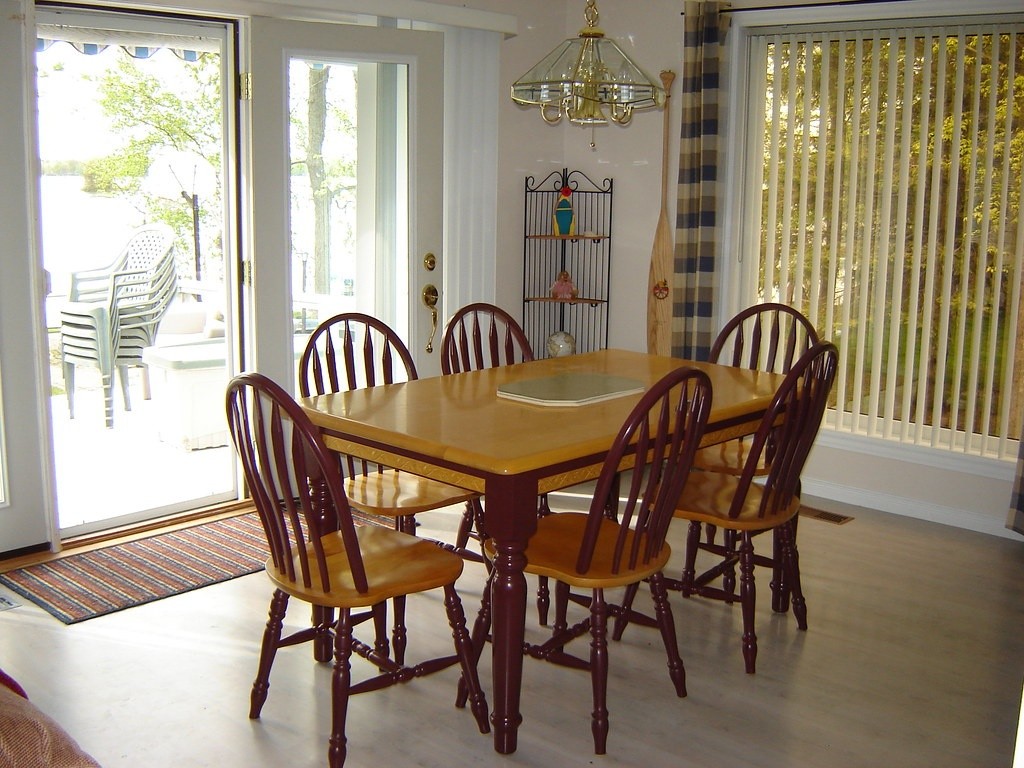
[276,349,814,754]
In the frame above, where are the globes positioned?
[546,331,575,358]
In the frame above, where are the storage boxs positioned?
[143,338,228,455]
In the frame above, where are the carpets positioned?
[1,501,421,626]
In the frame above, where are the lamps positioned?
[510,0,667,147]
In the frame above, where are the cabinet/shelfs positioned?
[522,168,614,363]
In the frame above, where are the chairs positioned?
[613,302,839,673]
[456,365,714,754]
[298,301,551,664]
[226,372,491,767]
[60,222,178,429]
